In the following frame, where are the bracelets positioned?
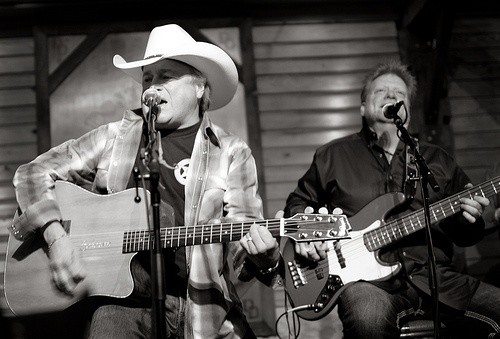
[47,231,68,251]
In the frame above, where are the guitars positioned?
[278,173,500,323]
[1,179,354,319]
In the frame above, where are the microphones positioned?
[142,89,161,106]
[382,101,404,119]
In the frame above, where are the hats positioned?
[113,24,238,111]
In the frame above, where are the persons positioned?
[283,61,500,339]
[11,22,280,339]
